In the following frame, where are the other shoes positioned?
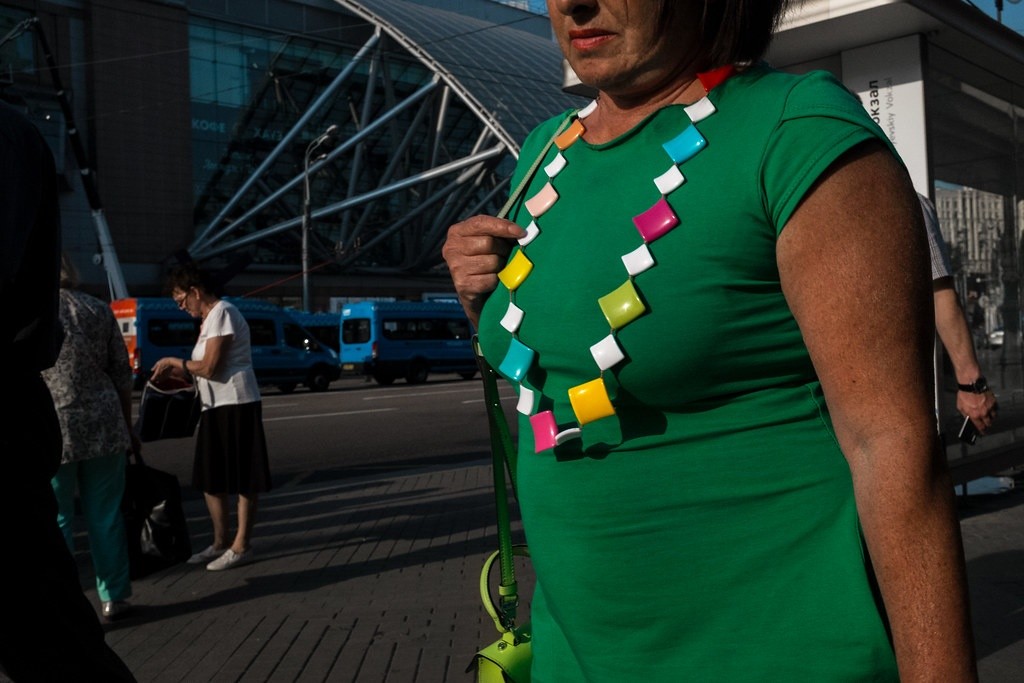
[101,599,112,616]
[187,545,227,563]
[206,549,248,571]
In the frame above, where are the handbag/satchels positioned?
[129,359,203,443]
[464,626,532,683]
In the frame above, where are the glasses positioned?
[173,290,193,308]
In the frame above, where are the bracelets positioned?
[183,360,189,374]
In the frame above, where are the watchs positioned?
[957,376,987,394]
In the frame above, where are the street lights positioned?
[303,125,340,315]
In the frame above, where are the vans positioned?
[110,296,341,393]
[338,301,483,385]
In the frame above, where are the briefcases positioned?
[938,408,1024,663]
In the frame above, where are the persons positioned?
[918,195,998,436]
[0,103,133,682]
[149,267,274,571]
[41,263,133,619]
[442,0,978,682]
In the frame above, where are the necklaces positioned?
[495,63,734,455]
